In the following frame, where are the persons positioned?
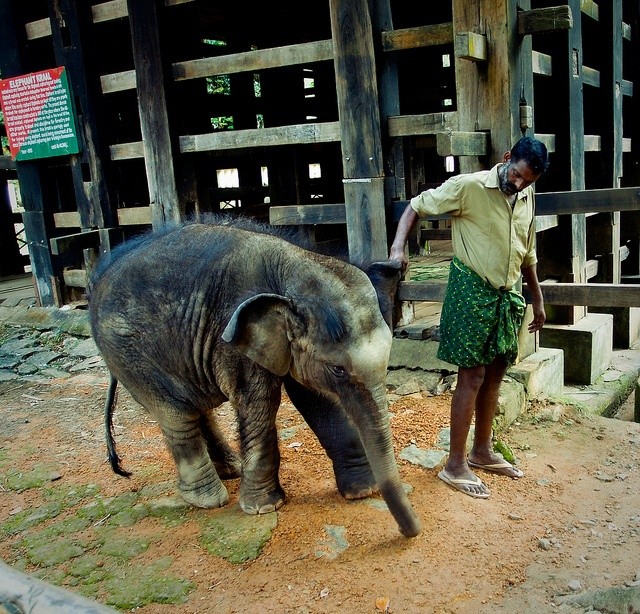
[389,136,545,497]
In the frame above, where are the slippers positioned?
[467,453,523,477]
[437,469,491,498]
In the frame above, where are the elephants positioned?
[87,212,423,538]
